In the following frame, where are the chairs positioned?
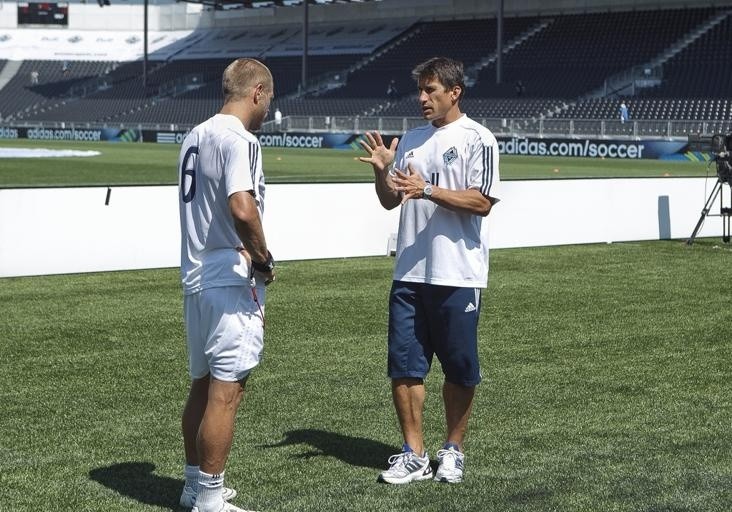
[0,0,731,142]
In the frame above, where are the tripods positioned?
[687,179,732,246]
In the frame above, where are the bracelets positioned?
[250,250,275,272]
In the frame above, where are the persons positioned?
[275,108,282,131]
[358,58,502,485]
[177,57,275,512]
[619,100,628,120]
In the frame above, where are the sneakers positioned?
[435,445,466,483]
[380,453,434,485]
[179,484,247,512]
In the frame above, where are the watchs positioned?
[423,184,433,200]
